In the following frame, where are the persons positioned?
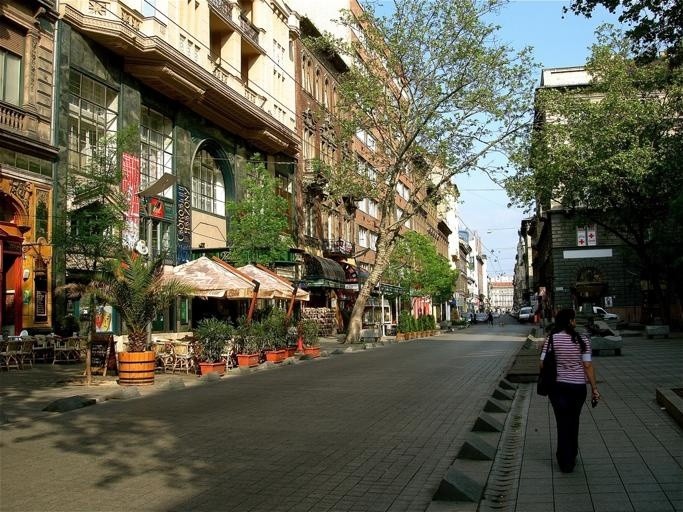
[539,308,600,473]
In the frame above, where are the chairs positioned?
[0,330,89,374]
[123,332,245,377]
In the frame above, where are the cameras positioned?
[590,396,598,408]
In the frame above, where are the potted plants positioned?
[90,252,194,388]
[393,309,437,342]
[191,309,323,385]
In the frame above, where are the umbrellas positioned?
[161,253,311,321]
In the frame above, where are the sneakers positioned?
[557,458,578,474]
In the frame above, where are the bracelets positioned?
[592,387,597,390]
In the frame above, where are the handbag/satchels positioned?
[536,332,558,396]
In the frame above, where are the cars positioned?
[460,306,534,322]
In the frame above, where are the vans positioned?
[578,305,619,320]
